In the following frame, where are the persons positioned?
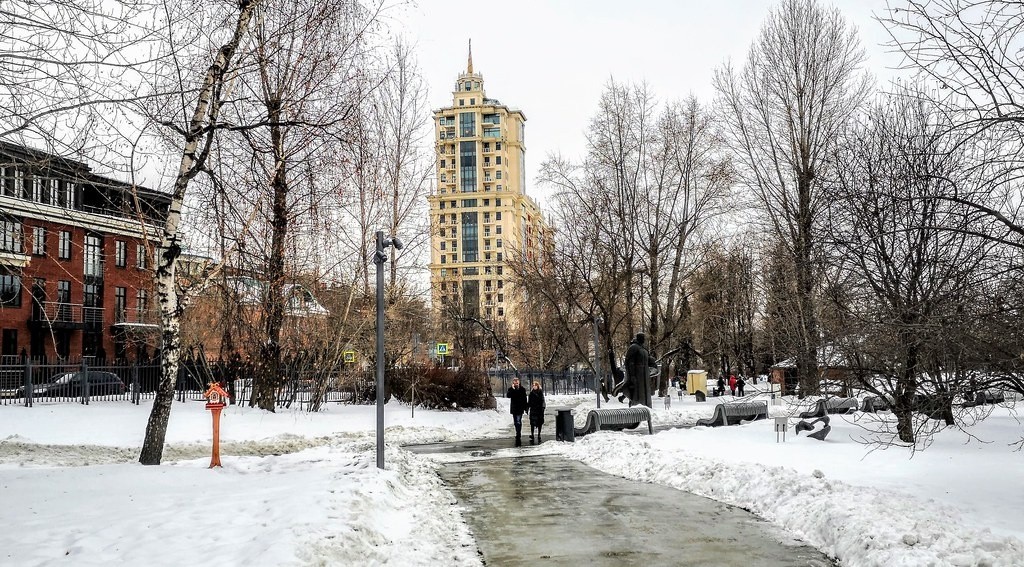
[624,332,653,409]
[526,381,546,439]
[670,375,687,391]
[736,376,745,397]
[717,376,726,396]
[729,375,737,396]
[507,378,528,439]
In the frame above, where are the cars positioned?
[15,371,125,397]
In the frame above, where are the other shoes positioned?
[538,436,541,439]
[529,435,534,439]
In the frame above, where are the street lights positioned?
[593,306,604,408]
[373,230,404,470]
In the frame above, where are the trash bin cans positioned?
[554,407,575,443]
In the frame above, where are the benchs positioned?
[799,398,859,418]
[963,392,1004,407]
[574,406,653,438]
[860,396,895,412]
[696,400,768,427]
